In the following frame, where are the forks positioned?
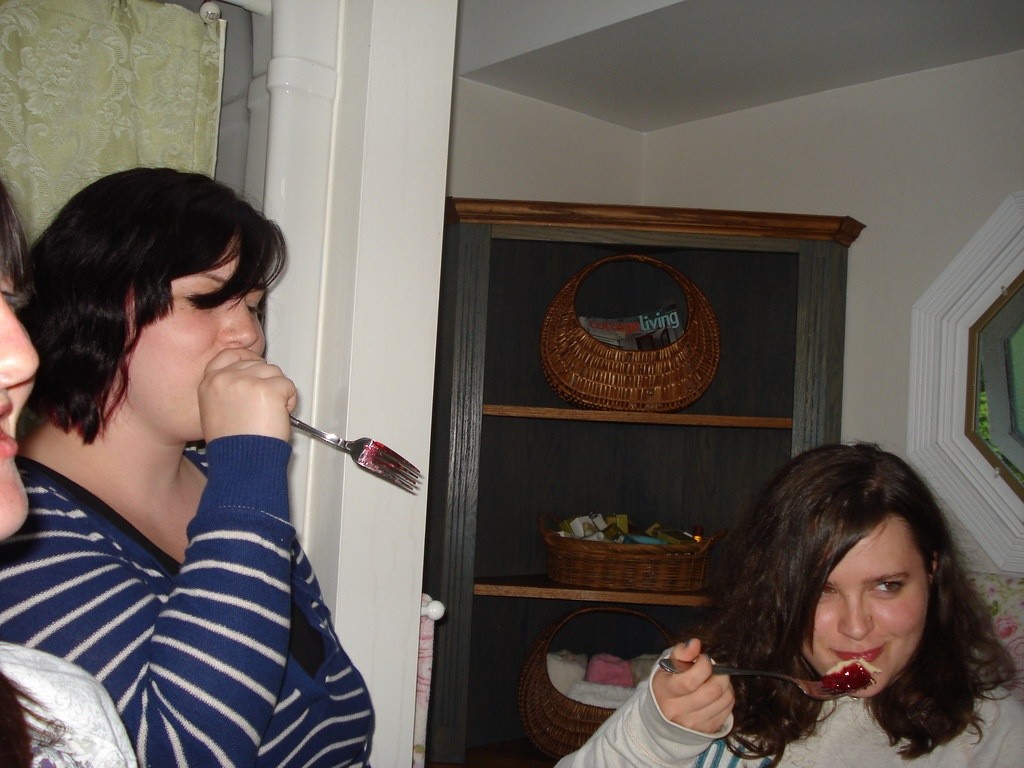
[657,657,875,701]
[287,414,425,497]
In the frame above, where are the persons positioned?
[0,179,138,768]
[0,168,375,768]
[555,441,1024,768]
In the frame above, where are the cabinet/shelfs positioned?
[424,197,867,768]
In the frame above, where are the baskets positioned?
[516,605,677,760]
[538,252,722,413]
[536,509,729,594]
